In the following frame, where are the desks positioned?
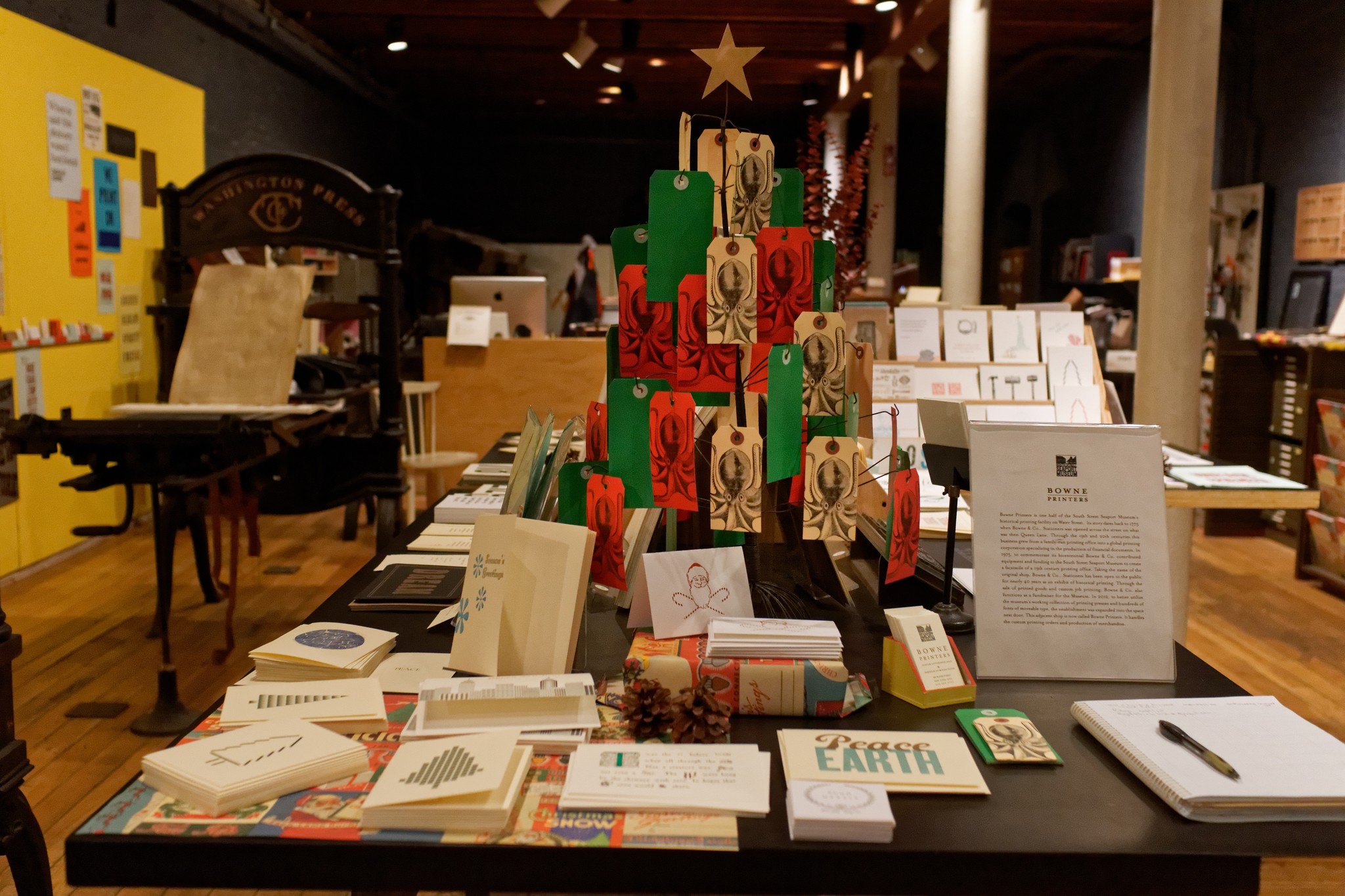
[54,423,1343,895]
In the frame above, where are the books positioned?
[76,263,779,857]
[896,230,1345,577]
[1072,690,1345,825]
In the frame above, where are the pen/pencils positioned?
[1158,720,1241,779]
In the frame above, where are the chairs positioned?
[375,373,484,530]
